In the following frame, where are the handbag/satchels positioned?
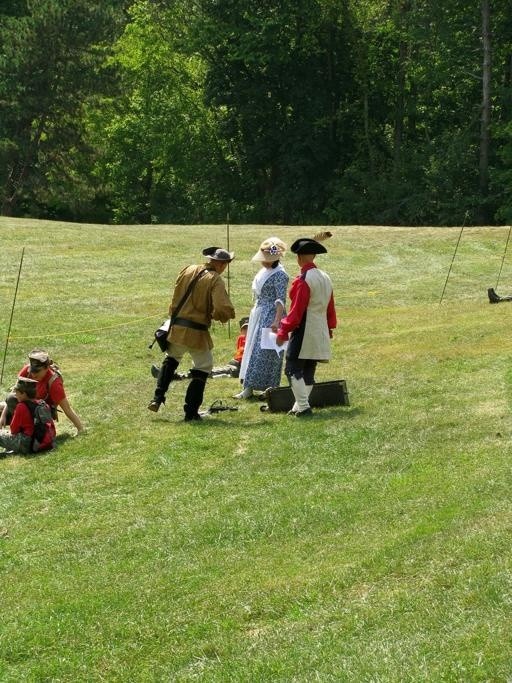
[155,319,171,352]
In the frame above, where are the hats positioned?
[252,237,288,261]
[201,246,236,261]
[290,238,327,254]
[9,350,49,390]
[239,317,249,331]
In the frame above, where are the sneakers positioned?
[150,364,160,378]
[184,412,201,421]
[288,408,312,418]
[148,400,159,412]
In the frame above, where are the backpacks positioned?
[48,361,64,422]
[19,400,56,452]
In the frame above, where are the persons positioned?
[0,374,57,456]
[0,346,85,438]
[275,237,339,418]
[149,316,250,381]
[231,235,290,400]
[145,244,236,423]
